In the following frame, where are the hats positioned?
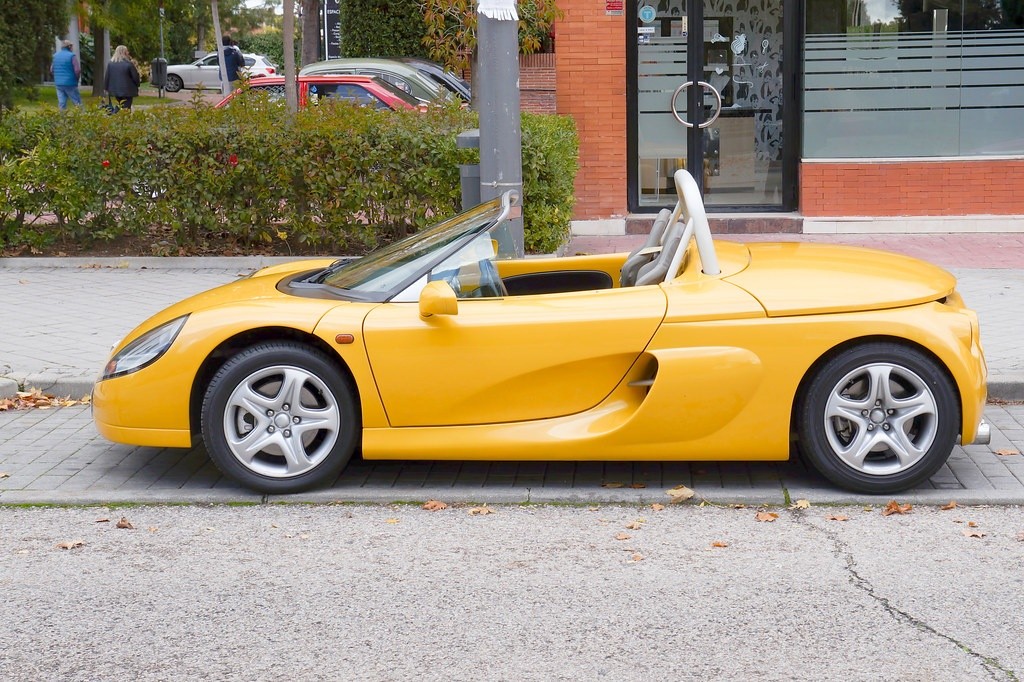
[60,40,75,48]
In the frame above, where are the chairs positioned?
[634,221,689,287]
[620,208,671,287]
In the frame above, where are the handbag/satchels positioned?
[101,95,115,116]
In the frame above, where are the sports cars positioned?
[88,170,991,492]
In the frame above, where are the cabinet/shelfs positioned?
[638,16,734,107]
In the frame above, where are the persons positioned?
[230,39,243,57]
[217,35,245,98]
[50,40,82,109]
[103,45,140,111]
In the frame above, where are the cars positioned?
[158,7,166,58]
[297,57,459,107]
[390,55,472,106]
[210,77,472,114]
[166,53,280,90]
